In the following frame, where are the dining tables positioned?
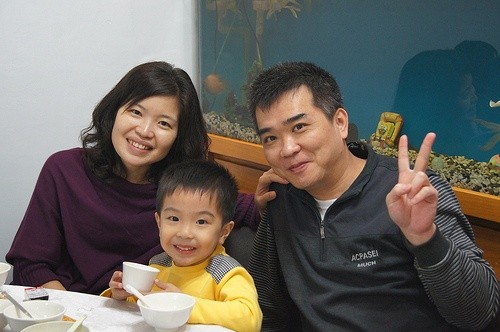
[1,285,237,332]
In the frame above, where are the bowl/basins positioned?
[3,300,67,332]
[0,300,13,329]
[0,262,11,288]
[137,293,195,332]
[20,320,91,332]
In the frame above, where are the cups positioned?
[123,261,161,295]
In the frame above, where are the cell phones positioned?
[24,286,49,301]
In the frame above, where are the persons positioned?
[245,61,500,332]
[4,61,289,296]
[97,157,265,332]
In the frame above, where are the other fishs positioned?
[474,118,500,151]
[201,73,231,96]
